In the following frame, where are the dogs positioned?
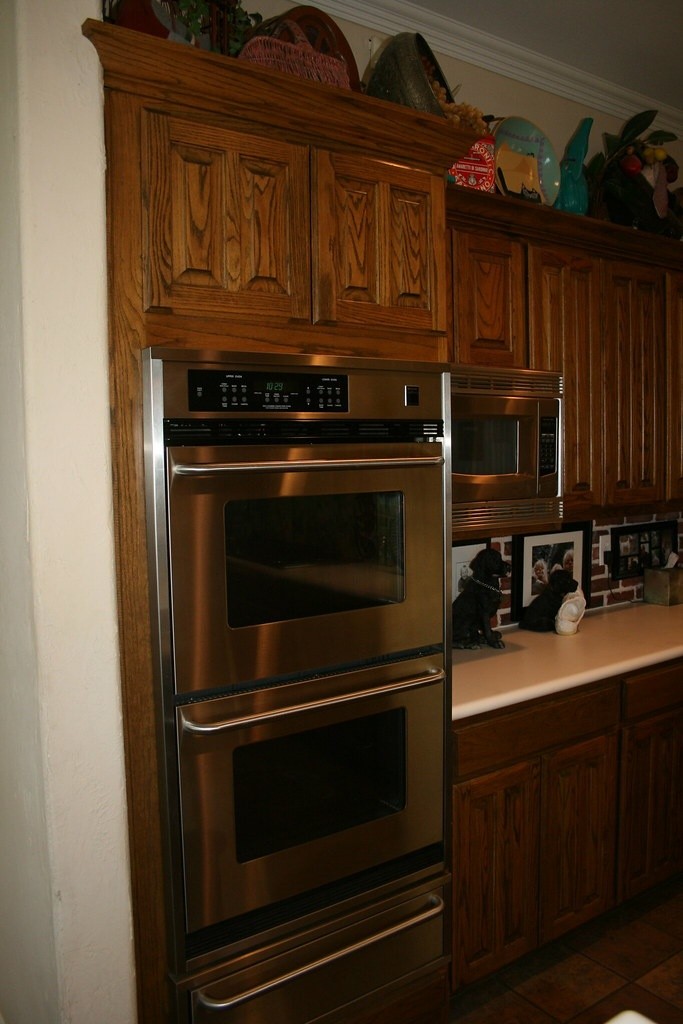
[453,549,507,648]
[518,571,577,633]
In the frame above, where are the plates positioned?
[492,117,560,206]
[449,132,498,193]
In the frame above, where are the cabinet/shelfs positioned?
[618,654,683,903]
[526,247,669,506]
[136,111,449,338]
[450,675,622,990]
[450,226,532,364]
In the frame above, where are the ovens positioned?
[142,345,458,1024]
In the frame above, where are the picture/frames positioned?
[452,540,492,604]
[510,522,596,612]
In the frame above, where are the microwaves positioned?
[448,365,564,531]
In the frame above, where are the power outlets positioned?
[599,535,612,566]
